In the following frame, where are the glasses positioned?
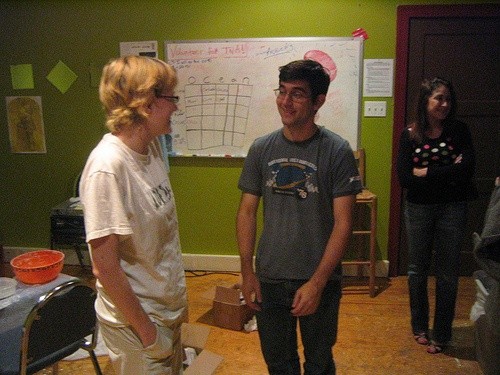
[273,87,310,101]
[159,94,179,99]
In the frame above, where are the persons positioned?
[234,60,363,375]
[396,76,477,354]
[80,56,188,374]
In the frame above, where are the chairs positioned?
[342,148,377,299]
[20,280,103,375]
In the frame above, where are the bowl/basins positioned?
[9,249,65,284]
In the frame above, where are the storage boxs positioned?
[181,323,224,375]
[213,283,258,330]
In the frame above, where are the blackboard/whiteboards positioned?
[165,37,362,158]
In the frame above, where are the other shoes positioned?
[415,333,427,343]
[428,345,444,352]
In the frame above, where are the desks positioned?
[0,274,81,374]
